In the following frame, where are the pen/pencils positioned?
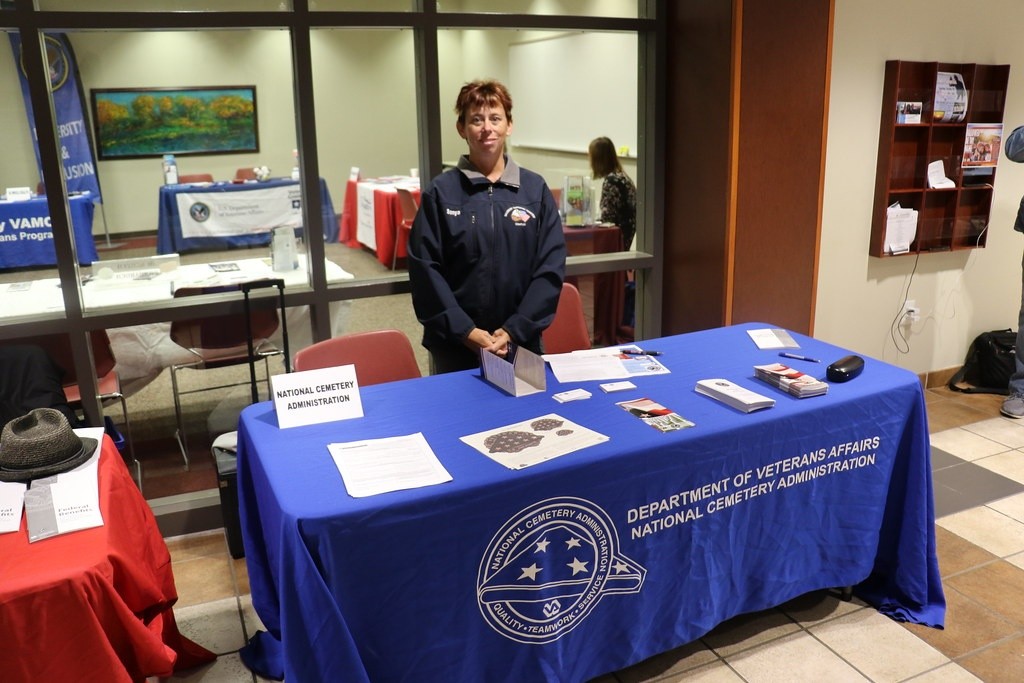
[623,349,664,356]
[778,352,821,363]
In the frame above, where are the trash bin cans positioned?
[81,417,126,463]
[210,431,245,560]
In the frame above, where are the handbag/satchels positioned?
[949,328,1017,395]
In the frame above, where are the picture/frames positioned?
[86,85,260,161]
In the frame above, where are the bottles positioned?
[162,154,179,188]
[292,148,301,180]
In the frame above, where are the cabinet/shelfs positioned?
[869,59,1014,258]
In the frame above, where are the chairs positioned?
[45,329,142,490]
[292,331,425,393]
[538,285,596,355]
[179,173,218,185]
[389,187,424,272]
[170,279,290,471]
[237,165,268,183]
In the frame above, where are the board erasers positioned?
[618,146,629,156]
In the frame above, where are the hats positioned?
[0,408,99,481]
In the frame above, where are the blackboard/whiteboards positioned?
[507,31,638,159]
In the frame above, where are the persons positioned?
[906,104,915,114]
[970,143,992,161]
[588,136,637,251]
[406,81,566,374]
[1000,125,1024,419]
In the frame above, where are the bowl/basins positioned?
[256,170,271,182]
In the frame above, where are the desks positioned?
[550,225,627,347]
[339,174,440,269]
[157,173,337,251]
[0,191,98,274]
[0,427,209,683]
[0,258,354,411]
[231,322,947,682]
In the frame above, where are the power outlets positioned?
[900,301,917,325]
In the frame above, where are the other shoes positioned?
[1000,392,1024,419]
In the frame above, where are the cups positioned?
[410,168,418,178]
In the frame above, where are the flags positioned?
[9,32,100,203]
